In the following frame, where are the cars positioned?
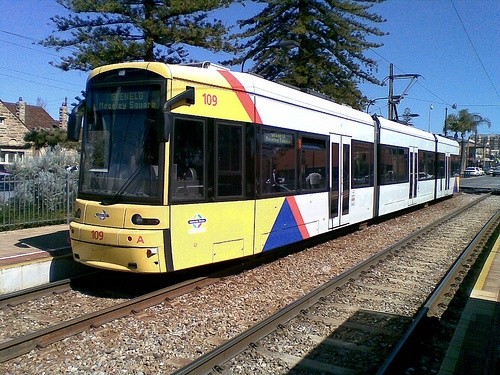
[464,165,500,177]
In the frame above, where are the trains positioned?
[67,60,461,274]
